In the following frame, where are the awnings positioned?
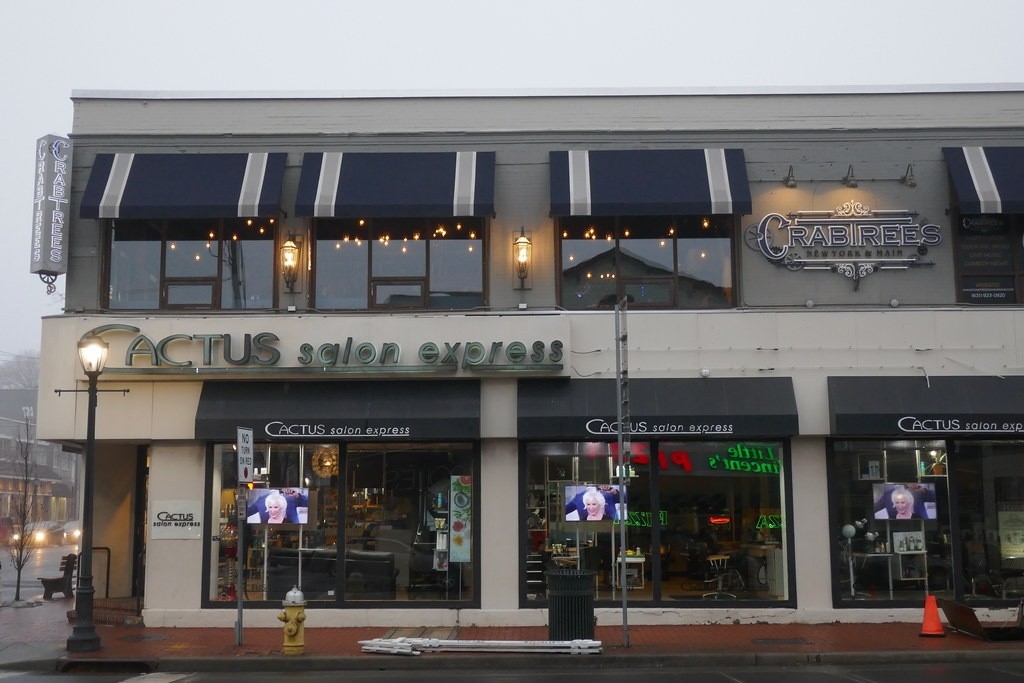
[516,377,800,438]
[82,148,752,217]
[827,376,1024,436]
[196,379,482,443]
[941,146,1024,214]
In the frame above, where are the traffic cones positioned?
[919,594,945,637]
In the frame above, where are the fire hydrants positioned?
[277,586,308,654]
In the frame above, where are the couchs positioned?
[271,546,399,600]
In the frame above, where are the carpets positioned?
[670,594,766,601]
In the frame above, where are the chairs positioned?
[697,532,743,600]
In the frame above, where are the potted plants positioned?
[922,449,946,474]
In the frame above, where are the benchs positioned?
[37,553,77,600]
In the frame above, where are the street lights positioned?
[54,328,130,653]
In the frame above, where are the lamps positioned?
[280,232,302,289]
[843,164,858,188]
[900,163,917,186]
[784,165,798,188]
[513,226,532,290]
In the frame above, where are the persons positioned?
[876,483,937,519]
[564,484,628,521]
[245,489,308,524]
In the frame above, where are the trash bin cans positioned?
[543,569,597,643]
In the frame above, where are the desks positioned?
[617,556,645,590]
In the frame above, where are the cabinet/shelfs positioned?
[435,491,449,601]
[849,441,950,600]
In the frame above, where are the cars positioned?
[9,520,80,547]
[316,538,425,598]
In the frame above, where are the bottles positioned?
[899,536,923,551]
[874,541,890,554]
[221,504,236,518]
[438,493,442,508]
[920,462,925,476]
[636,547,641,555]
